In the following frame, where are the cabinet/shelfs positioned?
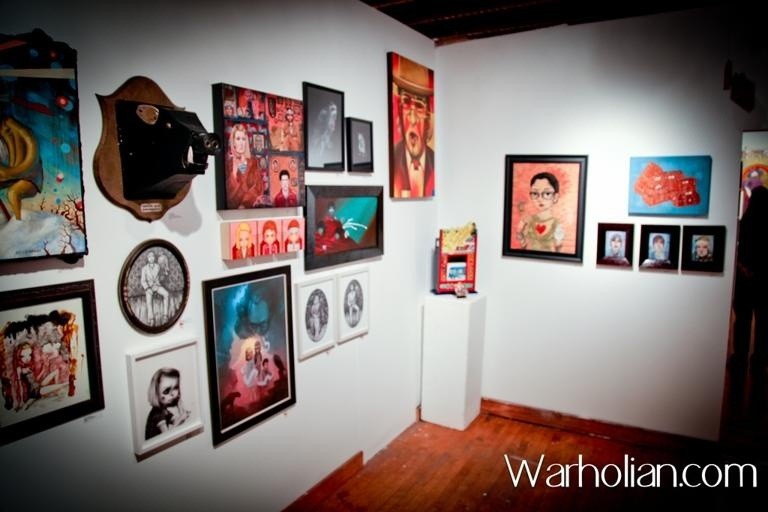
[420,290,488,431]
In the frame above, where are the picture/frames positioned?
[597,223,633,266]
[681,226,726,273]
[503,154,587,262]
[639,225,680,270]
